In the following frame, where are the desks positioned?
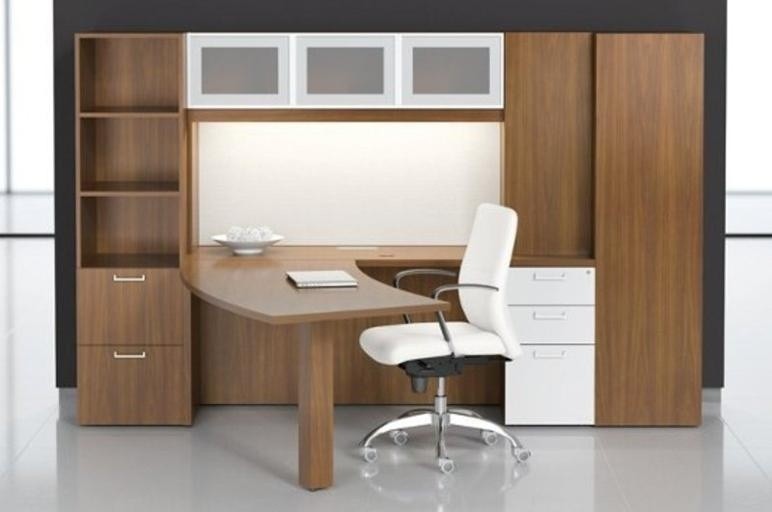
[180,244,505,492]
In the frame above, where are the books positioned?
[285,269,359,290]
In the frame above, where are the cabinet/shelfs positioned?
[188,34,506,109]
[74,34,188,269]
[77,269,195,426]
[504,266,595,428]
[597,33,704,429]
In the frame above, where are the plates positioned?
[207,233,284,256]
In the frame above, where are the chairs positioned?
[356,200,536,473]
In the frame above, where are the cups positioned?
[226,222,275,240]
